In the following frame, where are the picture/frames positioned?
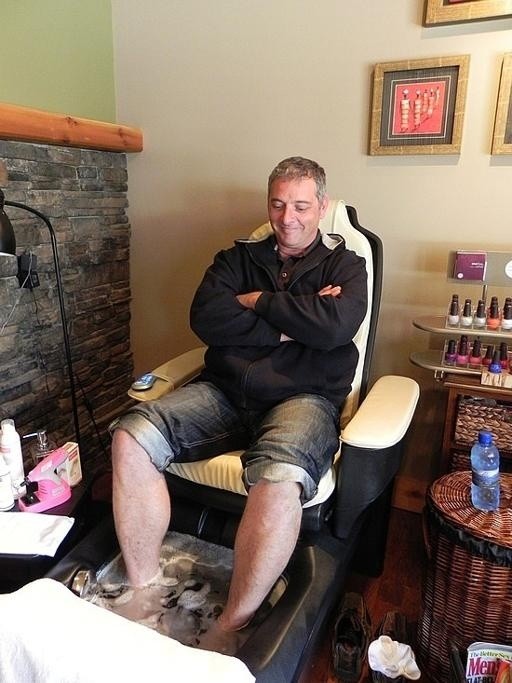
[366,0,512,156]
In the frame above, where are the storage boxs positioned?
[415,469,512,682]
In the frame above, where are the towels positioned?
[0,510,77,558]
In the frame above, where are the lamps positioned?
[0,187,90,443]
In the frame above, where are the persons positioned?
[112,158,368,655]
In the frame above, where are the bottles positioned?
[469,431,500,509]
[0,416,29,511]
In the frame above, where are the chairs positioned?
[125,199,421,580]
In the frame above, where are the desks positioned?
[0,465,98,593]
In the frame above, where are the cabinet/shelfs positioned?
[409,314,512,479]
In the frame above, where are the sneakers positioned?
[370,609,411,682]
[331,592,371,682]
